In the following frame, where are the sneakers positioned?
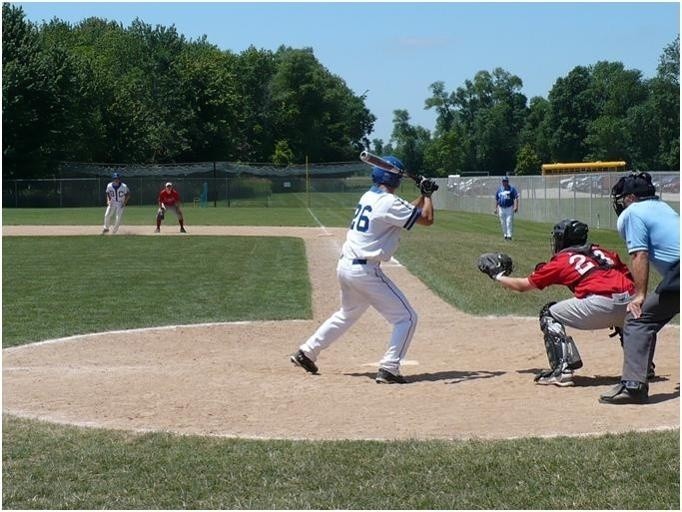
[181,228,186,233]
[375,367,403,384]
[290,349,318,375]
[599,381,650,404]
[534,368,576,387]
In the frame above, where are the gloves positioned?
[415,175,436,198]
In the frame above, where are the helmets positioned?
[372,157,405,188]
[610,172,658,219]
[165,182,172,189]
[501,176,509,183]
[549,218,589,255]
[112,172,120,179]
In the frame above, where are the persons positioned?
[598,171,680,404]
[478,219,655,387]
[102,174,131,233]
[290,156,435,383]
[494,176,519,241]
[154,182,186,232]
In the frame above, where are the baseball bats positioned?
[360,151,439,191]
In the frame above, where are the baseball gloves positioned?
[476,253,513,279]
